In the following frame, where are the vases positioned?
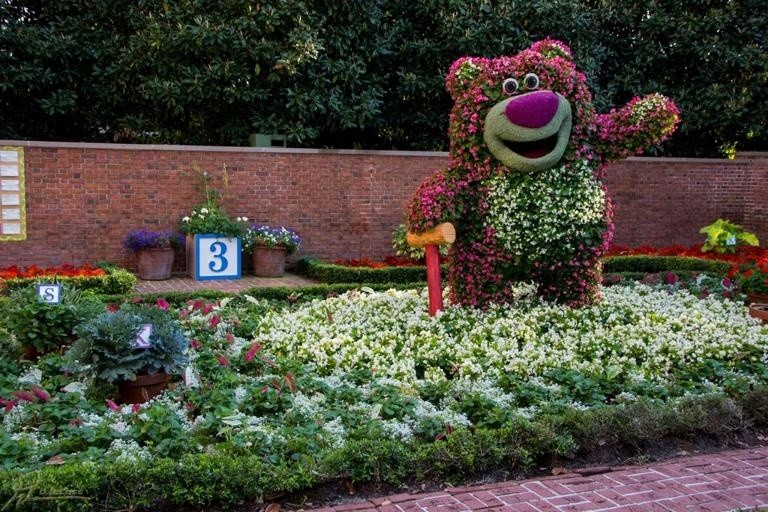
[251,243,289,277]
[135,247,175,279]
[185,232,242,280]
[748,292,768,324]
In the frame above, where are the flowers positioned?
[121,225,186,258]
[177,161,252,238]
[242,223,303,257]
[727,250,768,296]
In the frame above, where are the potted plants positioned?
[66,306,190,405]
